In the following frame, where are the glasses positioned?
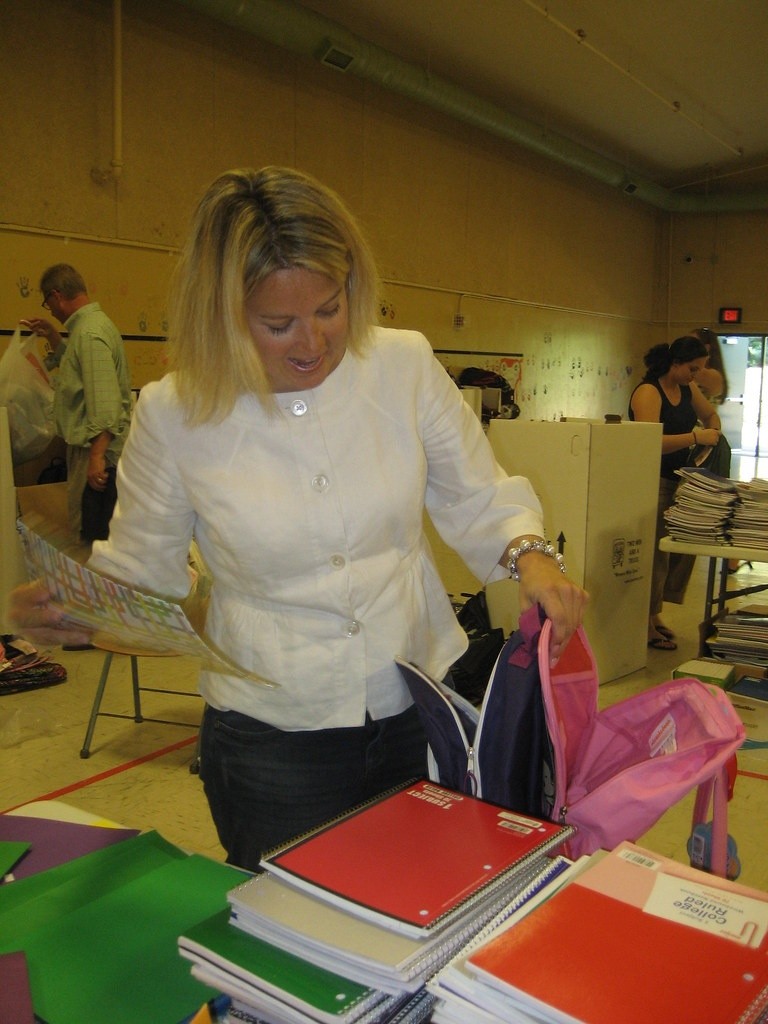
[702,327,709,348]
[41,288,60,310]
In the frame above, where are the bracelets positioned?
[508,538,567,582]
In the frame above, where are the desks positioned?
[0,799,236,1024]
[657,534,768,660]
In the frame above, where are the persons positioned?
[19,265,131,542]
[11,164,589,874]
[628,326,732,648]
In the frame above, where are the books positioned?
[176,777,767,1023]
[664,466,768,668]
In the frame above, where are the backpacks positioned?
[394,601,554,818]
[686,428,731,478]
[536,615,747,878]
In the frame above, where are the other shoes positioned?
[61,642,95,651]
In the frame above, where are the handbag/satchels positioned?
[0,319,57,466]
[81,466,118,543]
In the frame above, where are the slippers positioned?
[647,638,678,650]
[653,625,674,638]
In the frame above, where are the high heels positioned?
[719,561,753,575]
